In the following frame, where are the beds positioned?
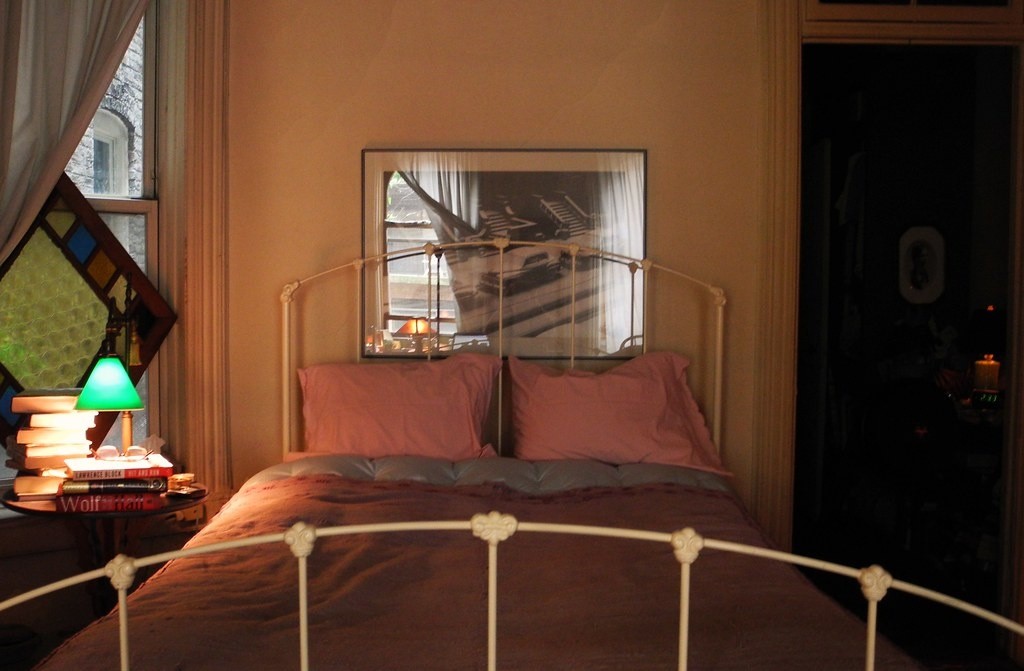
[0,235,1024,671]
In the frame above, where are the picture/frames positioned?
[359,147,647,356]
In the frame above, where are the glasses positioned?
[90,445,154,462]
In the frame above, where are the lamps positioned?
[75,296,146,456]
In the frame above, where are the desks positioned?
[0,482,210,615]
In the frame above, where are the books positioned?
[53,450,174,510]
[5,391,99,503]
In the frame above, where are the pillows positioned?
[298,351,506,462]
[508,348,733,476]
